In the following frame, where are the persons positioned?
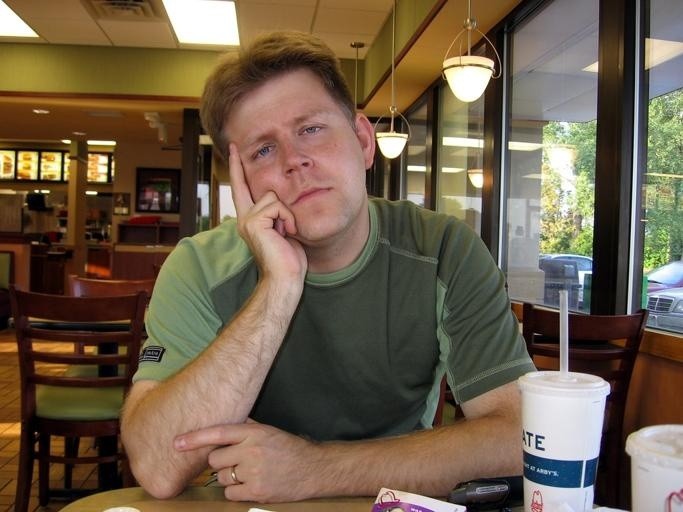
[118,31,541,501]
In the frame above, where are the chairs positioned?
[8,274,156,512]
[522,302,649,511]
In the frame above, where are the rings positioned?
[230,466,240,485]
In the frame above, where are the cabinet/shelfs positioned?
[111,218,182,283]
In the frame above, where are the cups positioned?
[519,369,611,509]
[626,424,682,510]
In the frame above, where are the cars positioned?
[539,254,593,308]
[647,260,683,333]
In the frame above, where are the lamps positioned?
[374,0,412,159]
[467,80,485,188]
[441,0,502,104]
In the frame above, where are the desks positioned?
[56,468,611,512]
